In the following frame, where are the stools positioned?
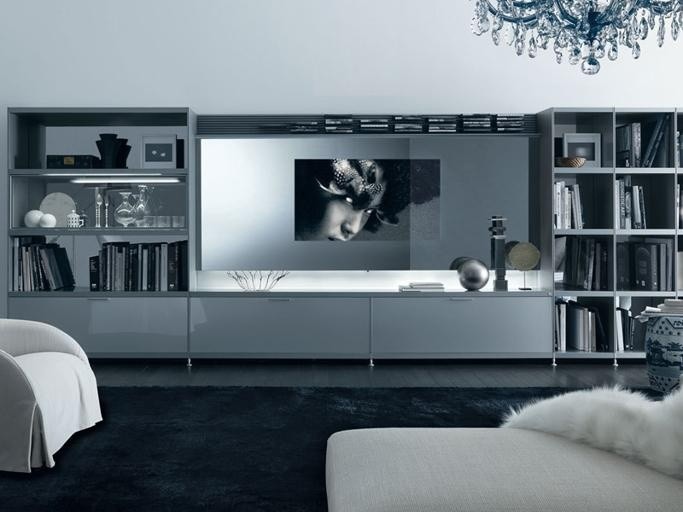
[324,426,683,512]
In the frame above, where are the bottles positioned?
[112,185,150,225]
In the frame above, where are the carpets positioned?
[0,386,682,512]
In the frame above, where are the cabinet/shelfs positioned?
[5,105,196,375]
[541,108,683,372]
[193,291,557,371]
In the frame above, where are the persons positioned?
[295,159,399,242]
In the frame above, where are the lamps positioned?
[471,1,683,76]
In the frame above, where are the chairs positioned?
[1,315,104,474]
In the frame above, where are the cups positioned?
[144,215,185,228]
[66,208,84,228]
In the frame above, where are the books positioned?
[554,114,683,352]
[11,236,188,291]
